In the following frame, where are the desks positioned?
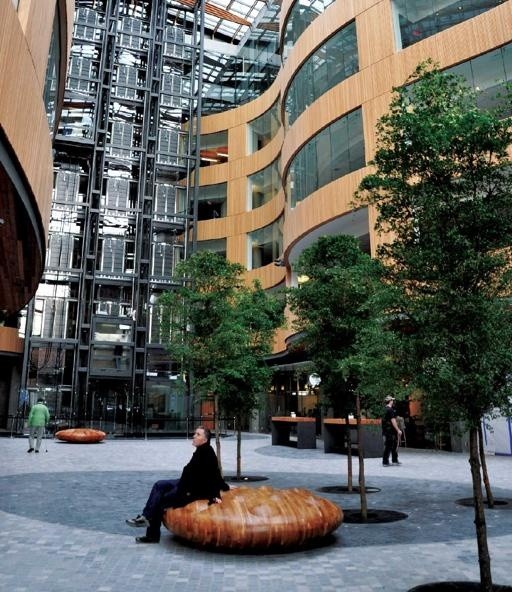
[271,416,383,459]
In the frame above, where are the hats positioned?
[385,395,395,401]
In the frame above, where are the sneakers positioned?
[384,462,402,466]
[135,536,160,543]
[35,450,39,452]
[125,515,149,527]
[28,448,34,452]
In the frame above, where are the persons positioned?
[26,397,51,453]
[125,425,238,544]
[381,394,403,467]
